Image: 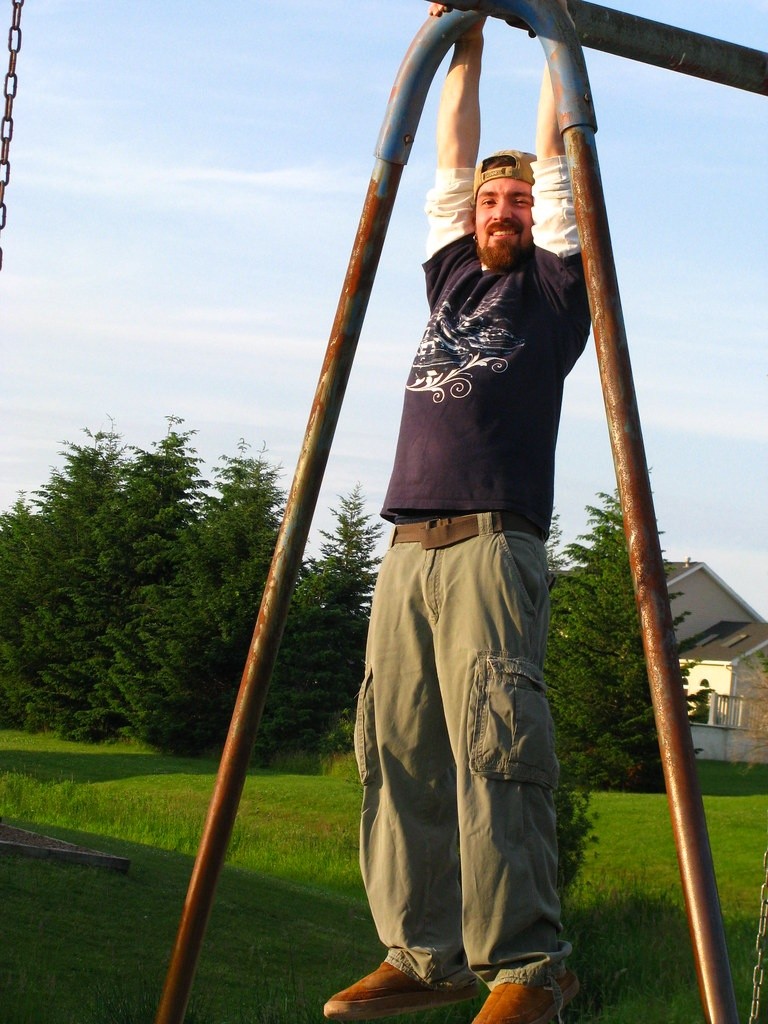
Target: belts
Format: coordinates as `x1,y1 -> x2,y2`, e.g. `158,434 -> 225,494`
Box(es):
392,516 -> 544,550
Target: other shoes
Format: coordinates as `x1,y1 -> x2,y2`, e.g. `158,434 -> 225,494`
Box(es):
471,967 -> 580,1024
324,963 -> 479,1020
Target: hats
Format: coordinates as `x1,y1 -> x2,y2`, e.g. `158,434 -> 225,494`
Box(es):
472,150 -> 537,204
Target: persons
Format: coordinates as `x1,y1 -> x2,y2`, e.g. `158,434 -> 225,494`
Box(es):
324,0 -> 591,1024
